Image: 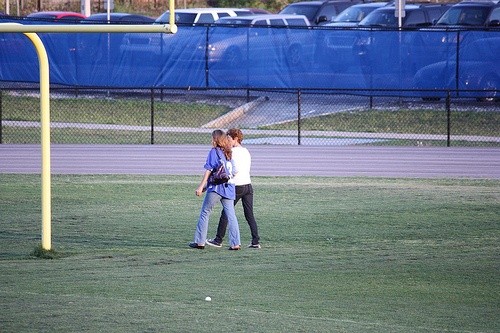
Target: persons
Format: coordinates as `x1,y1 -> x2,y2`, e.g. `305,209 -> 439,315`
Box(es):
188,130 -> 241,250
205,128 -> 261,248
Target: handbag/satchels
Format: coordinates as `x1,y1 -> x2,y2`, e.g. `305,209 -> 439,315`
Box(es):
209,148 -> 230,186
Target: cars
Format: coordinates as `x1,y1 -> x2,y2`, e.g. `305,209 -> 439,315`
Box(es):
120,8 -> 274,67
419,0 -> 500,102
80,13 -> 156,66
1,12 -> 86,64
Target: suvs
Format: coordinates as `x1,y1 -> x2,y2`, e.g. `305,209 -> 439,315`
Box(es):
171,14 -> 313,70
278,0 -> 453,72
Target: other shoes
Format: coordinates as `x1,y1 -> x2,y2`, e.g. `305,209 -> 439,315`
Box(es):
247,243 -> 260,249
206,239 -> 223,247
189,242 -> 204,249
228,247 -> 241,250
